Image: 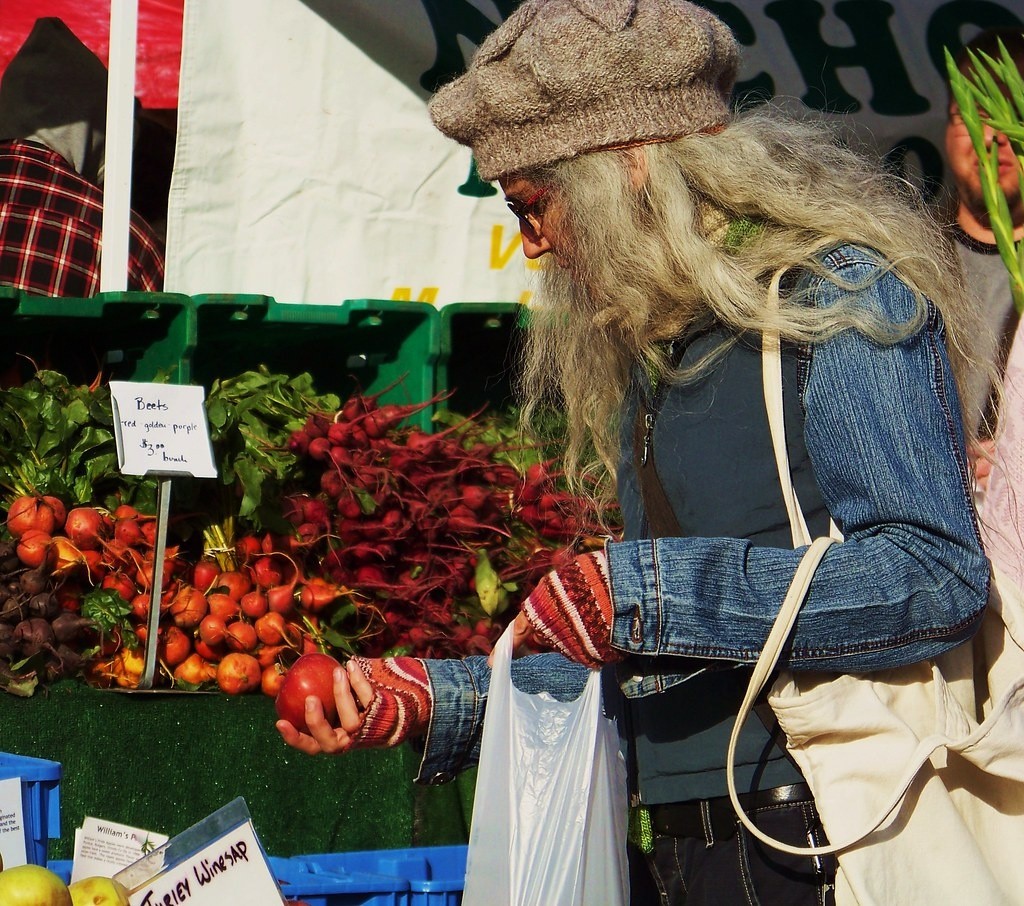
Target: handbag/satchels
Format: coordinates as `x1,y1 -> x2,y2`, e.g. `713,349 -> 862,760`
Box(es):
460,620 -> 631,906
767,609 -> 1024,906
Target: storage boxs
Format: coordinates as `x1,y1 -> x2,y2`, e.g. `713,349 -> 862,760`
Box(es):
0,751 -> 468,906
0,284 -> 575,442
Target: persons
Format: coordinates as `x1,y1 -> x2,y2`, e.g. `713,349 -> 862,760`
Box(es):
278,0 -> 993,905
925,0 -> 1024,519
0,17 -> 166,295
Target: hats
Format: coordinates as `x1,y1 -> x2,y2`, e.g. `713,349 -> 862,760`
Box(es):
428,0 -> 742,179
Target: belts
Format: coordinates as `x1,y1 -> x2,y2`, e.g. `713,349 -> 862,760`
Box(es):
650,782 -> 814,842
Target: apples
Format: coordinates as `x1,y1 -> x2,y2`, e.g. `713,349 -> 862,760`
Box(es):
275,653 -> 347,728
0,852 -> 128,906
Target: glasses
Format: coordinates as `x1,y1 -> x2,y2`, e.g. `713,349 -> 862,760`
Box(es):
507,181 -> 557,228
